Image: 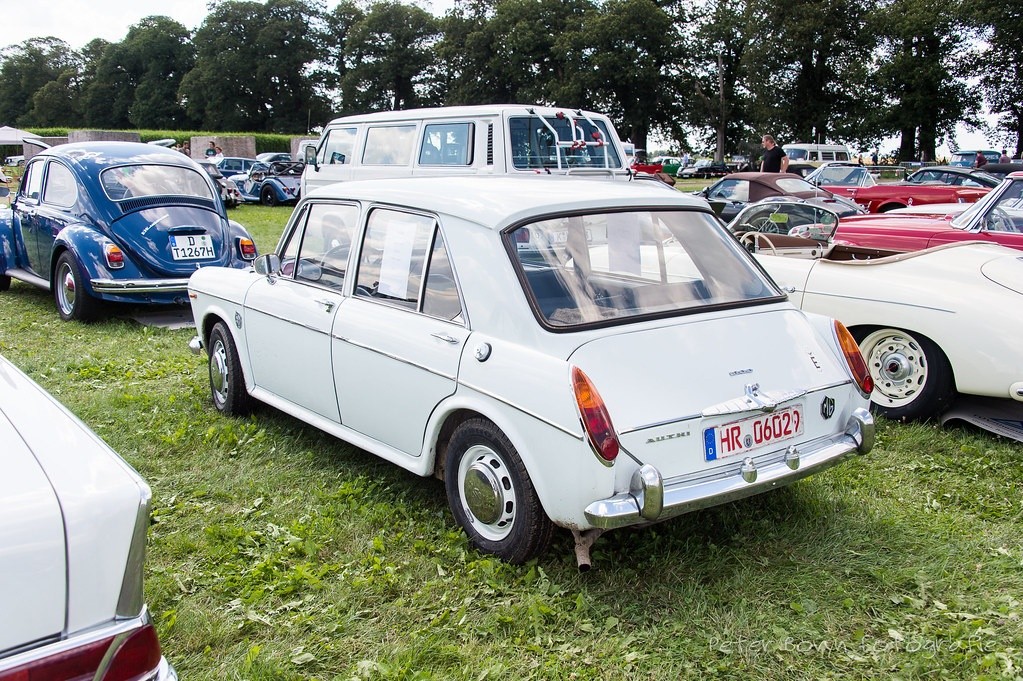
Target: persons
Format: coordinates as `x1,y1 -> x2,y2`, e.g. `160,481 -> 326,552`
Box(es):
681,150 -> 688,168
999,150 -> 1011,164
872,151 -> 881,179
974,149 -> 989,169
858,154 -> 865,168
759,135 -> 789,174
169,139 -> 226,160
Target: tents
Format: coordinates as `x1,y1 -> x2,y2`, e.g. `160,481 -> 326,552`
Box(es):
0,125 -> 44,167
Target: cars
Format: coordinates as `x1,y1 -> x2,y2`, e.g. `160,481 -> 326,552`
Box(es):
725,200 -> 1023,424
0,140 -> 261,321
620,140 -> 756,186
901,166 -> 1001,189
188,172 -> 875,560
803,160 -> 993,215
829,171 -> 1023,260
193,139 -> 321,209
685,172 -> 865,234
948,150 -> 1002,168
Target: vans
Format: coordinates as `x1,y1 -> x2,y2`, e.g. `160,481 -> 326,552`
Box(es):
300,105 -> 634,200
782,144 -> 850,169
